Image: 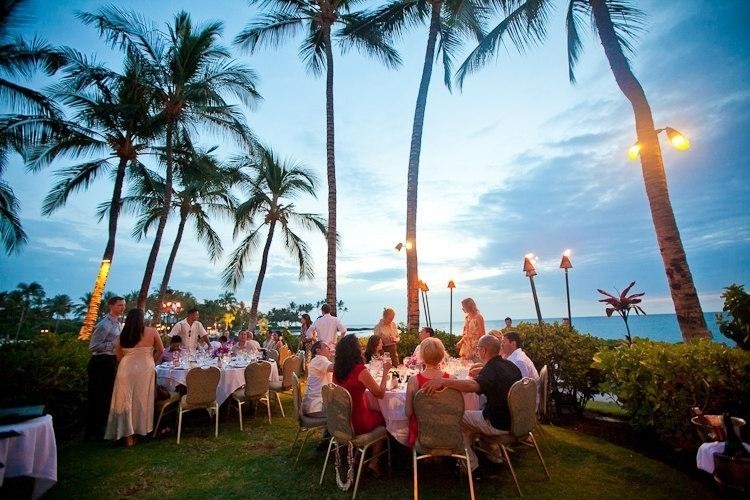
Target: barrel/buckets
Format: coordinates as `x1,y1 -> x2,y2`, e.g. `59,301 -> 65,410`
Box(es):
689,409 -> 746,444
4,404 -> 46,416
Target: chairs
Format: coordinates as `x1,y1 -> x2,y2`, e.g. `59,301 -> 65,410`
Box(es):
293,347 -> 549,500
154,348 -> 306,444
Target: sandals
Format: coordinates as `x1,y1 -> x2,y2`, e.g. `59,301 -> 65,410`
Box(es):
156,427 -> 171,436
124,435 -> 138,450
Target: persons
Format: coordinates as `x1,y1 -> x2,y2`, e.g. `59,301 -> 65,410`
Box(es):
87,296 -> 126,440
562,318 -> 569,327
103,308 -> 164,449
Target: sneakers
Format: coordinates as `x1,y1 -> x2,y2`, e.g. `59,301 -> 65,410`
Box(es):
473,466 -> 498,480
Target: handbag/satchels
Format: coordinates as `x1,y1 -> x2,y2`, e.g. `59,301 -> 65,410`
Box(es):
157,384 -> 171,402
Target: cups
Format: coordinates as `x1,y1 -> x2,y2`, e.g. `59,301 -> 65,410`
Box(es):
384,352 -> 390,366
157,347 -> 269,369
366,357 -> 474,388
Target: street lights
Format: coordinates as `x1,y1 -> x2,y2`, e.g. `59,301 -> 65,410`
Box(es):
519,248 -> 577,329
625,123 -> 692,159
393,241 -> 456,344
161,300 -> 183,328
